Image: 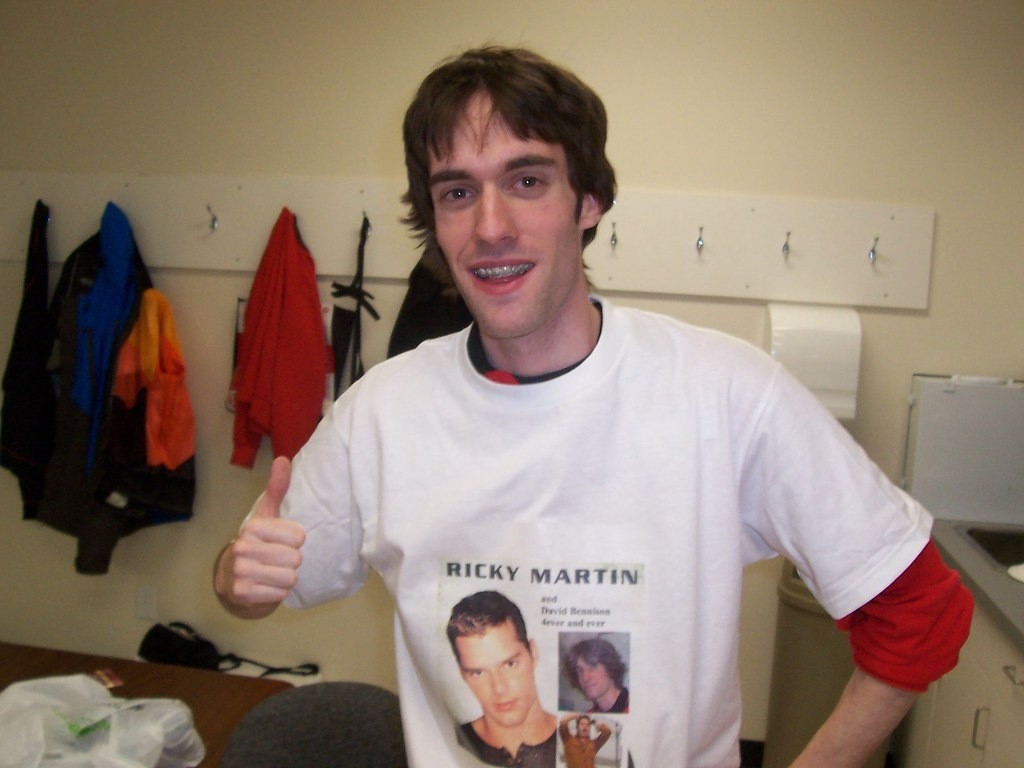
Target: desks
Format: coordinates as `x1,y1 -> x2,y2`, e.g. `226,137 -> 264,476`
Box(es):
1,643 -> 296,768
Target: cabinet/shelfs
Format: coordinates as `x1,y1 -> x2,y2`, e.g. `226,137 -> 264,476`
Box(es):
892,596 -> 1024,768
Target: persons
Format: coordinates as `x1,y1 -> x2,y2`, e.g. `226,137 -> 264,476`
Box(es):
209,43 -> 977,767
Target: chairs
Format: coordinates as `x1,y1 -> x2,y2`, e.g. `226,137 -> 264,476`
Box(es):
217,681 -> 409,768
